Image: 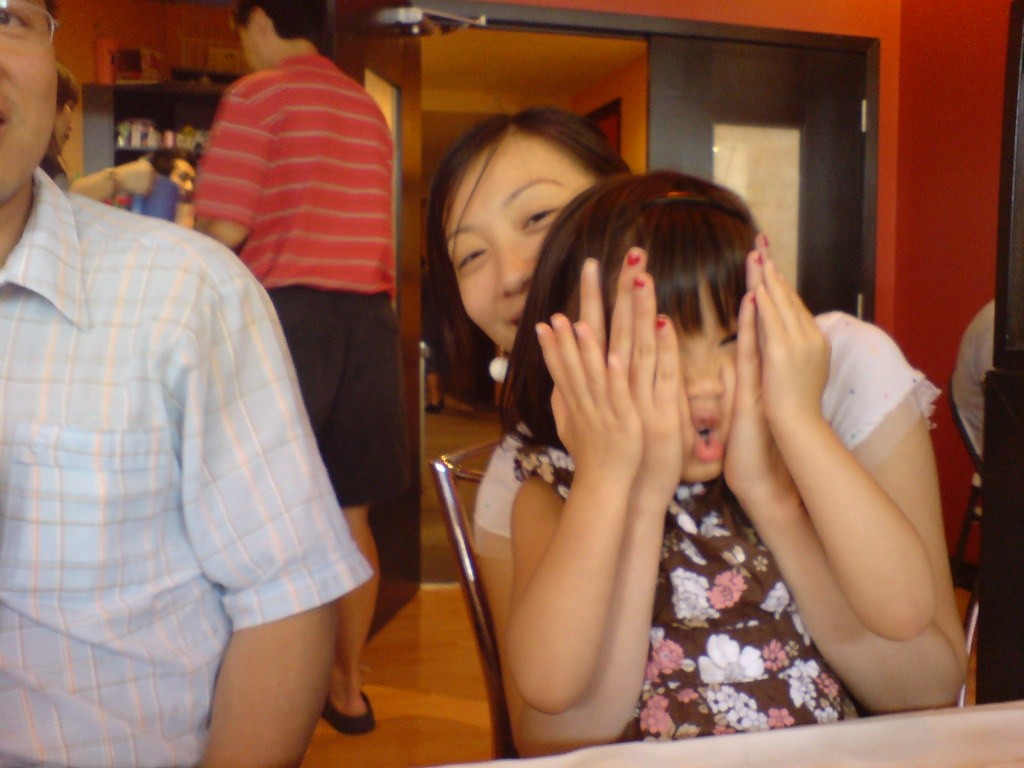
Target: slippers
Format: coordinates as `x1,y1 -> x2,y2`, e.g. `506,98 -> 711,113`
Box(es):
323,691 -> 376,733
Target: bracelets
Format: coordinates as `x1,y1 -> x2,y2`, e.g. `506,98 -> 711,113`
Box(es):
103,167 -> 119,198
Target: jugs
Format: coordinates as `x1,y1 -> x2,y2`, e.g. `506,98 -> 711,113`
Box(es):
131,175 -> 179,221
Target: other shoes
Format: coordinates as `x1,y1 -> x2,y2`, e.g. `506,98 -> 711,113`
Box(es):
425,403 -> 441,412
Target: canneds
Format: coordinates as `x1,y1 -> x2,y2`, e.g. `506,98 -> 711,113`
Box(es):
163,128 -> 177,148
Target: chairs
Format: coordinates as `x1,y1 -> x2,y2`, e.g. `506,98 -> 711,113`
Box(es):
430,374 -> 984,760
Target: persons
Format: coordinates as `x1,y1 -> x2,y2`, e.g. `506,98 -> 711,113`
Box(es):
420,105 -> 969,759
0,1 -> 376,768
39,59 -> 157,203
193,0 -> 408,736
154,146 -> 197,204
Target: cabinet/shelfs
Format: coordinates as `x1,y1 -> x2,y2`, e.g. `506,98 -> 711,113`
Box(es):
80,82 -> 229,228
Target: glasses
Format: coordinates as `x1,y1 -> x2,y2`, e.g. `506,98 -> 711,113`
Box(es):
0,1 -> 56,45
